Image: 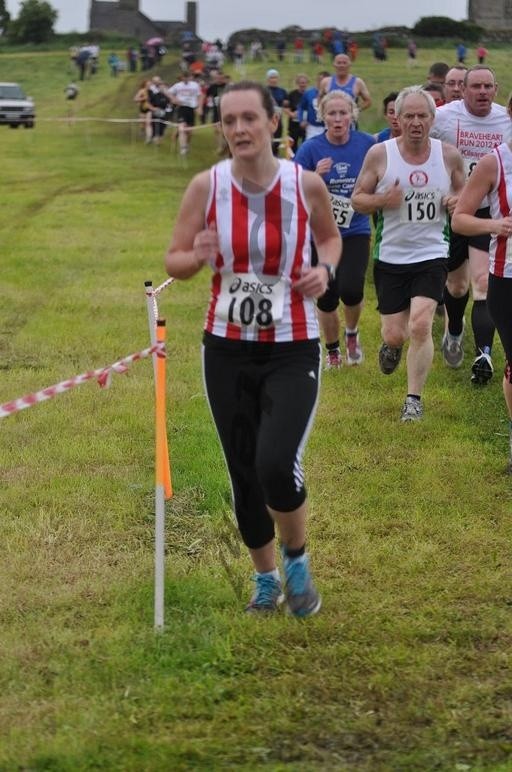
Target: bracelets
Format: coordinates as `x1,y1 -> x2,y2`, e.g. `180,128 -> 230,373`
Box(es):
317,262 -> 336,282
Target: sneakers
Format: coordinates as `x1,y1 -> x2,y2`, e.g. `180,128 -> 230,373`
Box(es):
138,133 -> 195,159
325,341 -> 343,372
398,395 -> 424,427
282,542 -> 323,620
343,328 -> 363,367
470,353 -> 495,386
378,341 -> 402,375
442,316 -> 466,369
242,565 -> 285,617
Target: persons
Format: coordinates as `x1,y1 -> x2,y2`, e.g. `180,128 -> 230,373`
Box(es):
422,62 -> 512,387
261,70 -> 287,156
63,78 -> 81,102
296,71 -> 332,140
292,90 -> 379,377
371,90 -> 403,143
287,73 -> 309,155
132,65 -> 236,158
181,34 -> 419,73
476,43 -> 488,64
453,39 -> 468,65
449,91 -> 512,417
68,37 -> 166,78
349,86 -> 466,426
163,80 -> 343,626
318,53 -> 372,131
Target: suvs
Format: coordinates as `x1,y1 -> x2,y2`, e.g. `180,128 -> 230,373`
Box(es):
0,81 -> 36,130
